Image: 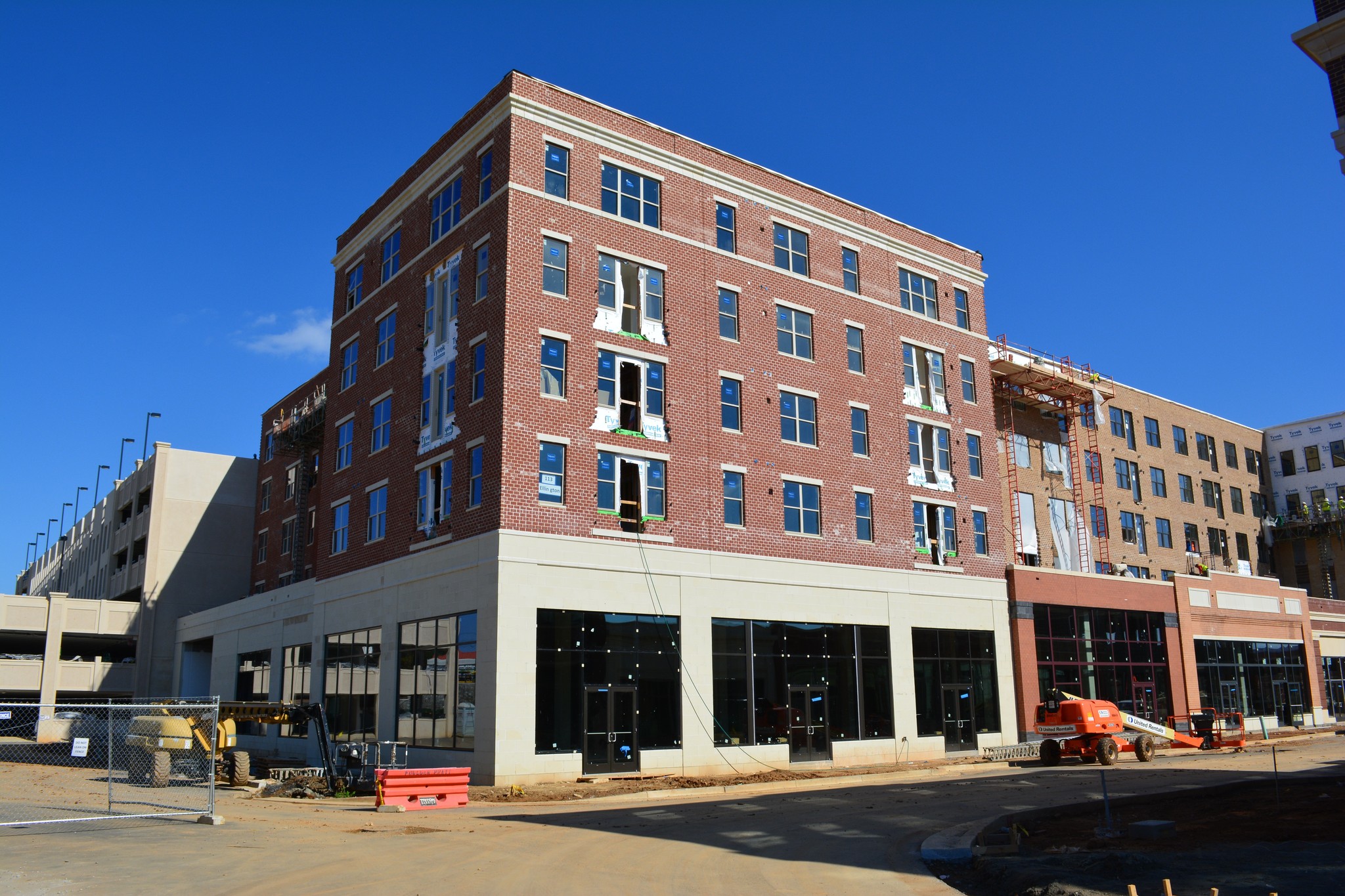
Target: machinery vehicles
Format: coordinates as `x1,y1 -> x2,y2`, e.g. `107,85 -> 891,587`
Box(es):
1033,688 -> 1246,767
124,699 -> 408,793
